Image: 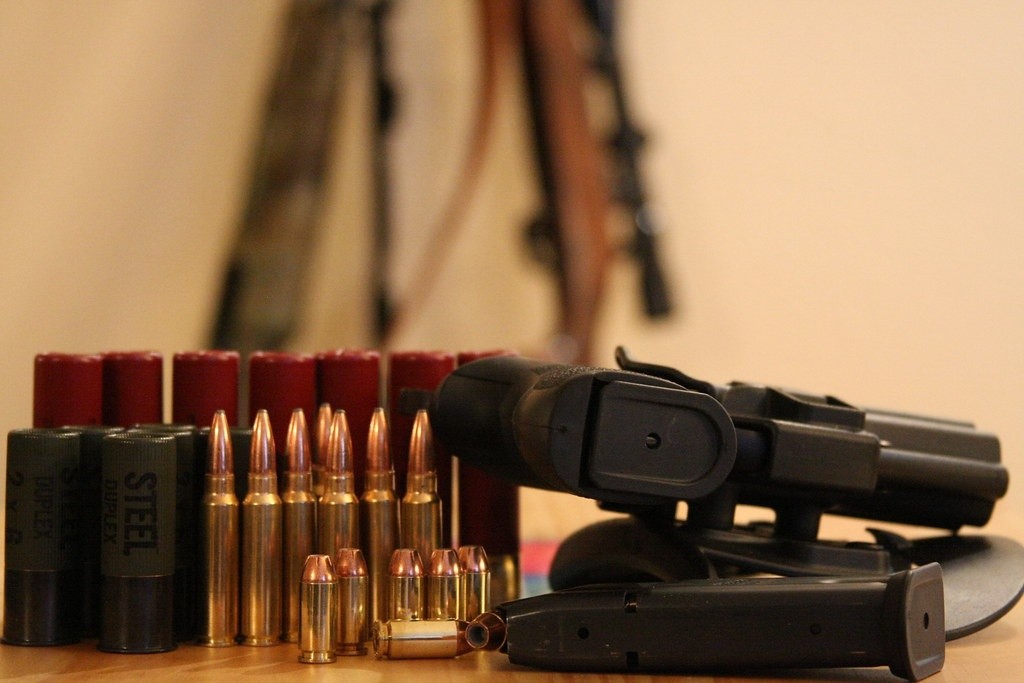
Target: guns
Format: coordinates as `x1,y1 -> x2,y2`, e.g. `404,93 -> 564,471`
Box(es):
200,0 -> 679,364
398,346 -> 1008,544
